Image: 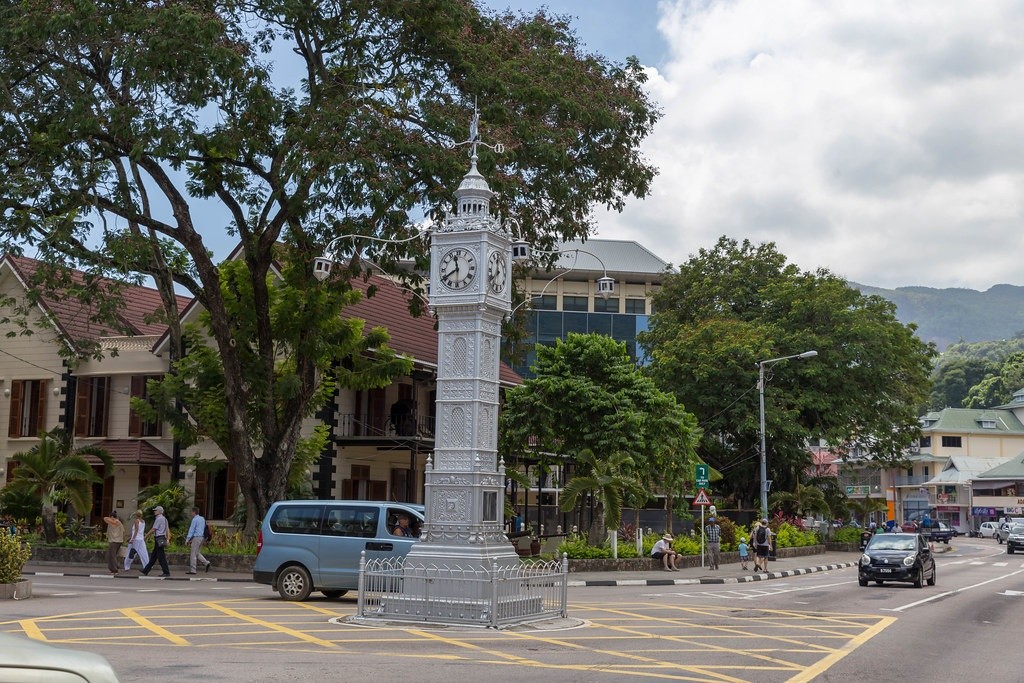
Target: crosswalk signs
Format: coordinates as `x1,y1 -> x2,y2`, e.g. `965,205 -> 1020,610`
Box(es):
692,489 -> 712,505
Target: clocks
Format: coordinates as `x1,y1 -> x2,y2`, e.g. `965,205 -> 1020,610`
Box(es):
487,250 -> 508,294
438,248 -> 477,289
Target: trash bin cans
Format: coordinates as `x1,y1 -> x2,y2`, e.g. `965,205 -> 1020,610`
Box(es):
860,532 -> 872,552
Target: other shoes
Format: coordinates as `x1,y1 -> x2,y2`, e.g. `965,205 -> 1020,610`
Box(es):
715,563 -> 718,569
671,567 -> 680,571
754,564 -> 769,573
158,573 -> 170,577
109,569 -> 119,574
139,569 -> 147,576
664,566 -> 672,572
205,564 -> 210,573
742,566 -> 748,570
709,566 -> 714,570
185,571 -> 196,574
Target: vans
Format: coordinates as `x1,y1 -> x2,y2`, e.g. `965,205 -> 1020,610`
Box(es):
252,500 -> 427,602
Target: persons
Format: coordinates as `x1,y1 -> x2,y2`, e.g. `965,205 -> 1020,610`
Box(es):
932,519 -> 939,528
185,507 -> 210,574
738,537 -> 750,570
753,519 -> 773,573
668,533 -> 682,569
123,510 -> 150,571
137,506 -> 171,577
881,522 -> 888,532
517,513 -> 522,532
104,512 -> 123,575
392,514 -> 415,538
749,522 -> 762,571
705,518 -> 721,570
891,522 -> 903,532
651,534 -> 680,571
922,513 -> 932,527
875,525 -> 884,534
912,518 -> 921,533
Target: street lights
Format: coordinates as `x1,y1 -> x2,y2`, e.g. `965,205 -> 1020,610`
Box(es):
760,350 -> 817,524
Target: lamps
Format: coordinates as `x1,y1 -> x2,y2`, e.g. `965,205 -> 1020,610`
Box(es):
119,468 -> 125,477
305,470 -> 311,477
0,468 -> 4,475
4,389 -> 10,396
123,386 -> 128,394
52,388 -> 59,396
187,469 -> 193,477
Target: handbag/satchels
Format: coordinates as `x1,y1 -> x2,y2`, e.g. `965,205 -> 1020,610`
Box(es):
129,548 -> 137,559
155,536 -> 167,547
117,547 -> 126,557
750,531 -> 754,547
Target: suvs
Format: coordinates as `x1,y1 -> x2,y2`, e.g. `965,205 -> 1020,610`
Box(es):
1006,524 -> 1024,554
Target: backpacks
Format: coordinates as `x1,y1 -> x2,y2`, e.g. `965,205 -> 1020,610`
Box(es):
202,516 -> 212,541
756,526 -> 767,544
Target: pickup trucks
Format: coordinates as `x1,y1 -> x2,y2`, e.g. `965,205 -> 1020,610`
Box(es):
995,522 -> 1021,544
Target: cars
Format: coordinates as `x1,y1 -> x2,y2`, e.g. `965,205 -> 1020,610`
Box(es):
814,519 -> 842,527
979,522 -> 999,539
858,532 -> 936,588
901,520 -> 954,544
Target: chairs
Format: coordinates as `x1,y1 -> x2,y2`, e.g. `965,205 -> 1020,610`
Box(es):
388,515 -> 398,532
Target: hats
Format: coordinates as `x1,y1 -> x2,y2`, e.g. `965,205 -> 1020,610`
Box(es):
762,519 -> 769,523
135,510 -> 143,517
709,517 -> 715,523
152,505 -> 164,513
398,513 -> 409,521
662,534 -> 673,541
740,537 -> 746,542
755,522 -> 762,527
